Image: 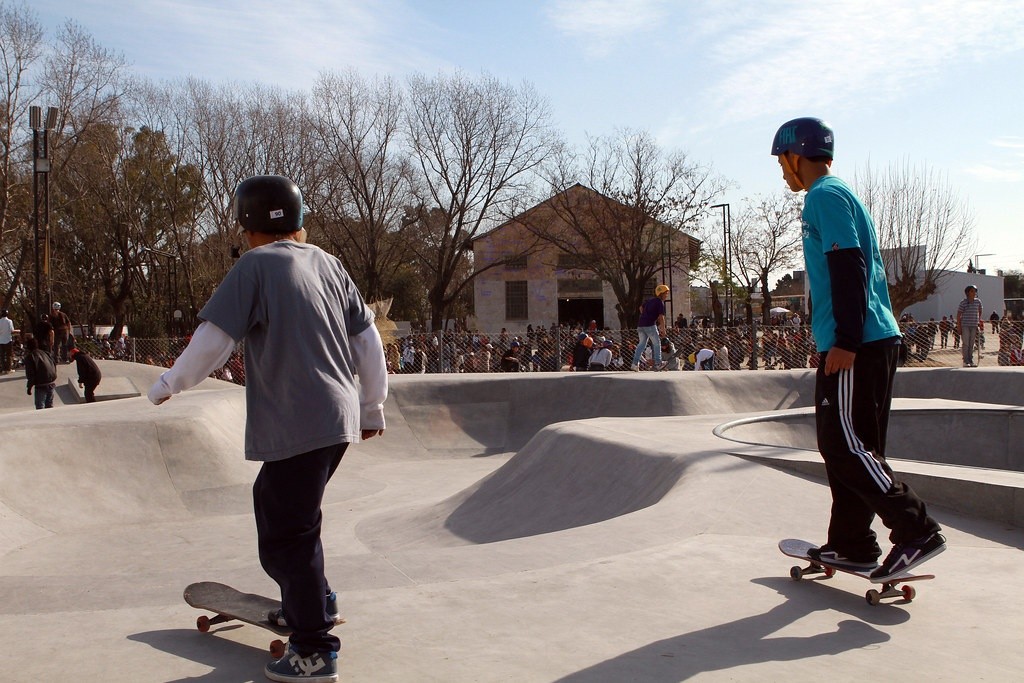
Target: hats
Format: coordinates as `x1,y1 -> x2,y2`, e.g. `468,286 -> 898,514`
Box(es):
592,320 -> 596,323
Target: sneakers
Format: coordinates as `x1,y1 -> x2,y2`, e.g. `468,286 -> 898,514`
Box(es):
264,650 -> 340,683
267,590 -> 339,625
806,540 -> 878,569
868,531 -> 947,584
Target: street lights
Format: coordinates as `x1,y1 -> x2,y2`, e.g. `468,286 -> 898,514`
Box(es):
29,106 -> 58,320
710,203 -> 735,328
975,254 -> 996,272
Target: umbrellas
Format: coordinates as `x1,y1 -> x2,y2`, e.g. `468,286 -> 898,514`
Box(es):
770,306 -> 790,313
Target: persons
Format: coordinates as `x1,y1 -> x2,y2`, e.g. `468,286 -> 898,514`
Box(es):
71,348 -> 102,403
898,312 -> 928,360
0,309 -> 21,374
147,176 -> 388,682
33,314 -> 54,353
11,334 -> 245,386
48,302 -> 71,364
771,117 -> 948,586
381,313 -> 820,373
990,310 -> 999,333
25,338 -> 57,410
631,285 -> 670,371
998,314 -> 1024,366
928,314 -> 985,350
957,285 -> 983,367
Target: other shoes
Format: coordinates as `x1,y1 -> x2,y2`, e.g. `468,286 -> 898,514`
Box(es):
1,369 -> 15,375
65,359 -> 70,363
963,362 -> 978,367
656,360 -> 669,370
631,364 -> 639,372
55,359 -> 60,363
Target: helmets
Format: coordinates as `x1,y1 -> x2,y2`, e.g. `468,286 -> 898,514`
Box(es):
583,336 -> 593,347
52,301 -> 63,311
233,175 -> 303,235
1,309 -> 9,316
511,341 -> 520,347
771,117 -> 834,163
485,344 -> 493,349
603,340 -> 613,348
70,348 -> 79,358
655,284 -> 670,297
660,337 -> 669,347
579,332 -> 589,340
688,352 -> 696,364
41,313 -> 48,320
965,285 -> 978,295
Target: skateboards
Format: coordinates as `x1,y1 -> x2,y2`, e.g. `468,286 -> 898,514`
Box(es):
654,345 -> 684,372
977,326 -> 984,366
778,538 -> 936,606
183,580 -> 348,657
